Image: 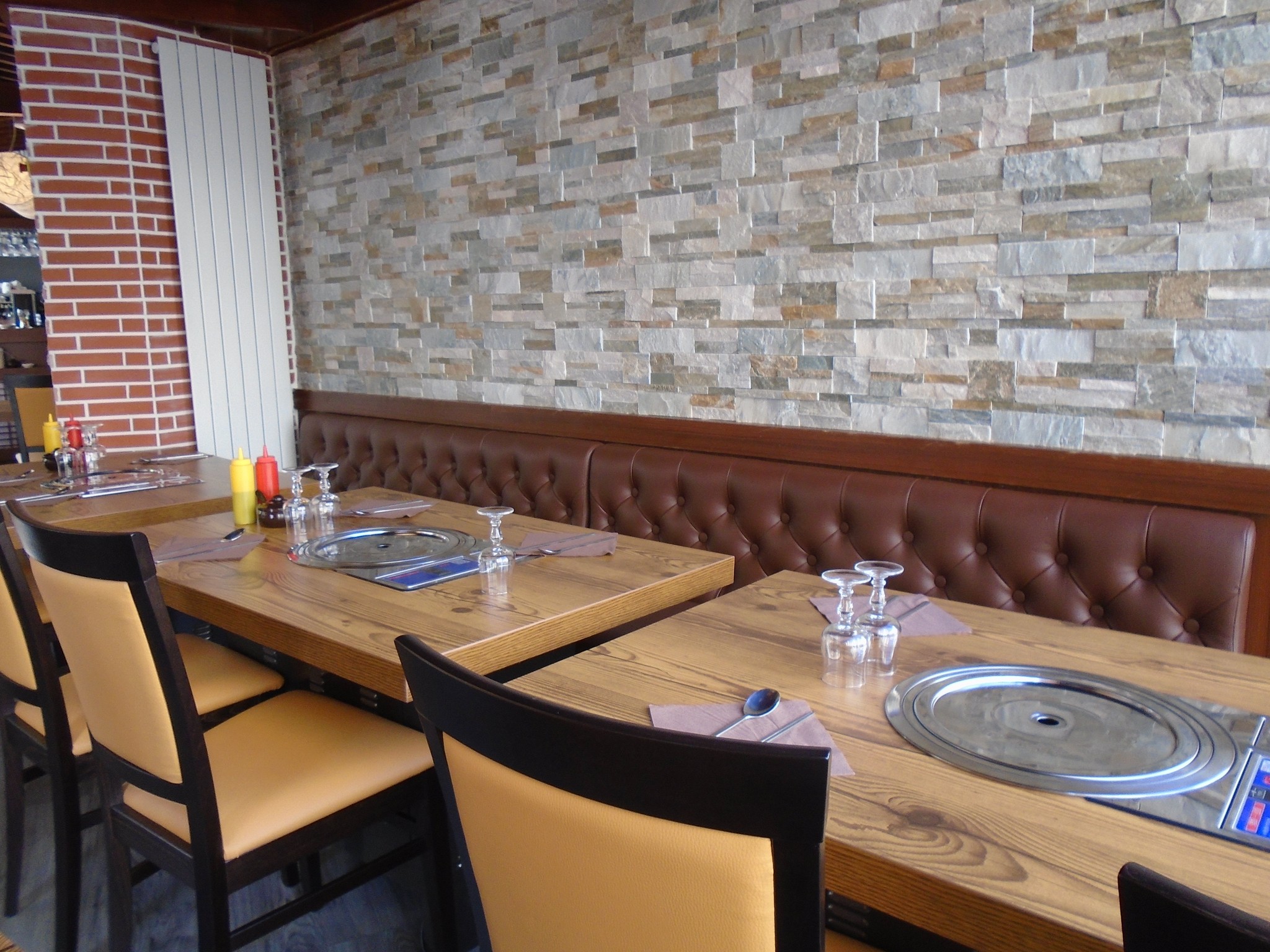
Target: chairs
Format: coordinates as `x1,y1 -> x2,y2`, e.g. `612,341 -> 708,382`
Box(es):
7,499 -> 462,952
2,374 -> 57,462
0,511 -> 284,951
396,634 -> 831,951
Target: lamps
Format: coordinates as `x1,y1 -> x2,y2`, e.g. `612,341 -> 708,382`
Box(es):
0,115 -> 33,205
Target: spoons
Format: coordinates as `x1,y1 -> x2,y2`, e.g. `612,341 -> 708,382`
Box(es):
14,469 -> 35,479
153,528 -> 246,556
711,688 -> 780,737
350,504 -> 432,516
538,537 -> 614,555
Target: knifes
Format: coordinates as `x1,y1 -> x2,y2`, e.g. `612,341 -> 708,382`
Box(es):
154,540 -> 260,563
515,532 -> 599,552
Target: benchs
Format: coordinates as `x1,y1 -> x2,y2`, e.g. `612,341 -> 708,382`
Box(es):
289,389 -> 1270,657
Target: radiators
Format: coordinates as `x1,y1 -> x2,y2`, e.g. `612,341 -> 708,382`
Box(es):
154,37 -> 298,472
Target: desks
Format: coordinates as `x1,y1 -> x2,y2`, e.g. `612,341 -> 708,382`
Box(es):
0,452 -> 322,550
129,485 -> 734,702
500,569 -> 1270,951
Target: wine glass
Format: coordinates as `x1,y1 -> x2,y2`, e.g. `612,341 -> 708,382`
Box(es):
477,506 -> 514,595
820,569 -> 871,688
309,463 -> 341,531
53,424 -> 105,478
852,560 -> 903,678
281,466 -> 312,536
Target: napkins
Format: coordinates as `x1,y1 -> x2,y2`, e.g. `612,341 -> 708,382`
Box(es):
647,698 -> 855,778
131,453 -> 214,465
3,490 -> 81,506
515,532 -> 619,556
341,500 -> 438,519
809,593 -> 972,636
0,474 -> 54,486
152,533 -> 267,561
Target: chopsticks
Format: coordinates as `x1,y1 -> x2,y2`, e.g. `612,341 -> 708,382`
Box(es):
760,711 -> 815,743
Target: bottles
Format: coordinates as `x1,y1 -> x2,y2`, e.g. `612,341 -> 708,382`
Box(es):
229,447 -> 257,525
42,413 -> 61,454
64,413 -> 82,450
255,445 -> 280,504
